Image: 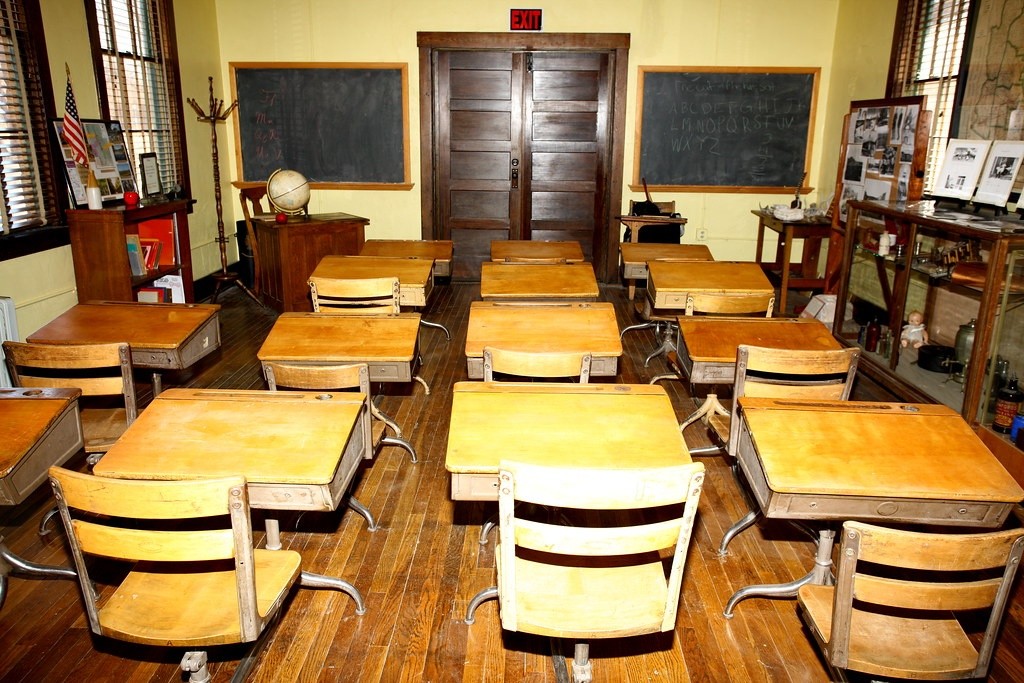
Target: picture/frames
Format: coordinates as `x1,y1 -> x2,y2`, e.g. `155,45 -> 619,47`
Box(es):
933,139 -> 992,200
973,139 -> 1024,207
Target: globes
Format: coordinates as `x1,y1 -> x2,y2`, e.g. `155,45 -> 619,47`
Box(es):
266,167 -> 310,223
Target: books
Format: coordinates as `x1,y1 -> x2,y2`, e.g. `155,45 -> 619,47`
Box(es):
125,234 -> 164,276
137,287 -> 172,303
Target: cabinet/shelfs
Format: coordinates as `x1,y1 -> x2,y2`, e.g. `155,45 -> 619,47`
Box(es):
65,198 -> 194,304
832,198 -> 1024,518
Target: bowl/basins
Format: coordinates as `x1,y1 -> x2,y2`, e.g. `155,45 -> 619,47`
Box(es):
918,344 -> 961,372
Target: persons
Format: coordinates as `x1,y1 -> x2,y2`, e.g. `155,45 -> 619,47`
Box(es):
900,311 -> 929,349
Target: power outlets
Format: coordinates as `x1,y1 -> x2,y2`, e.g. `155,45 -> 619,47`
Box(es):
697,229 -> 708,241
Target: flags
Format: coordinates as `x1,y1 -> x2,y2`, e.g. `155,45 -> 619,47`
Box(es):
62,76 -> 90,170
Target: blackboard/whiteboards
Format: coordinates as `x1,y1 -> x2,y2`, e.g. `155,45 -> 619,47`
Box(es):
229,61 -> 415,191
628,65 -> 822,195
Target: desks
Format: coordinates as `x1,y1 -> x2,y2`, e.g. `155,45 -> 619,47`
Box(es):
618,242 -> 714,338
722,396 -> 1024,620
492,239 -> 585,263
26,301 -> 222,398
645,261 -> 774,367
93,388 -> 367,615
480,261 -> 600,302
257,312 -> 422,439
306,255 -> 436,365
465,302 -> 622,379
359,239 -> 454,341
751,211 -> 830,314
671,315 -> 845,433
0,387 -> 102,610
258,210 -> 369,314
444,381 -> 695,683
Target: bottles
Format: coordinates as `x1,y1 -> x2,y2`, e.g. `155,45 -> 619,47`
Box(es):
878,230 -> 890,256
976,354 -> 1024,452
905,171 -> 924,210
896,173 -> 908,210
954,324 -> 975,383
857,314 -> 894,363
86,170 -> 98,187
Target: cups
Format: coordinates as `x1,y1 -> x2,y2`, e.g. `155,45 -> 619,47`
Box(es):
86,188 -> 102,209
889,234 -> 897,246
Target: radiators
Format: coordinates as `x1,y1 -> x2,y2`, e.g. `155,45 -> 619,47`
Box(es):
0,296 -> 18,388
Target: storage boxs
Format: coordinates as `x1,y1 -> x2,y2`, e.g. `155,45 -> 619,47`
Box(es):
815,295 -> 853,324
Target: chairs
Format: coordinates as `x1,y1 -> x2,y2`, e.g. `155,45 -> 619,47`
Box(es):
0,185 -> 1024,683
